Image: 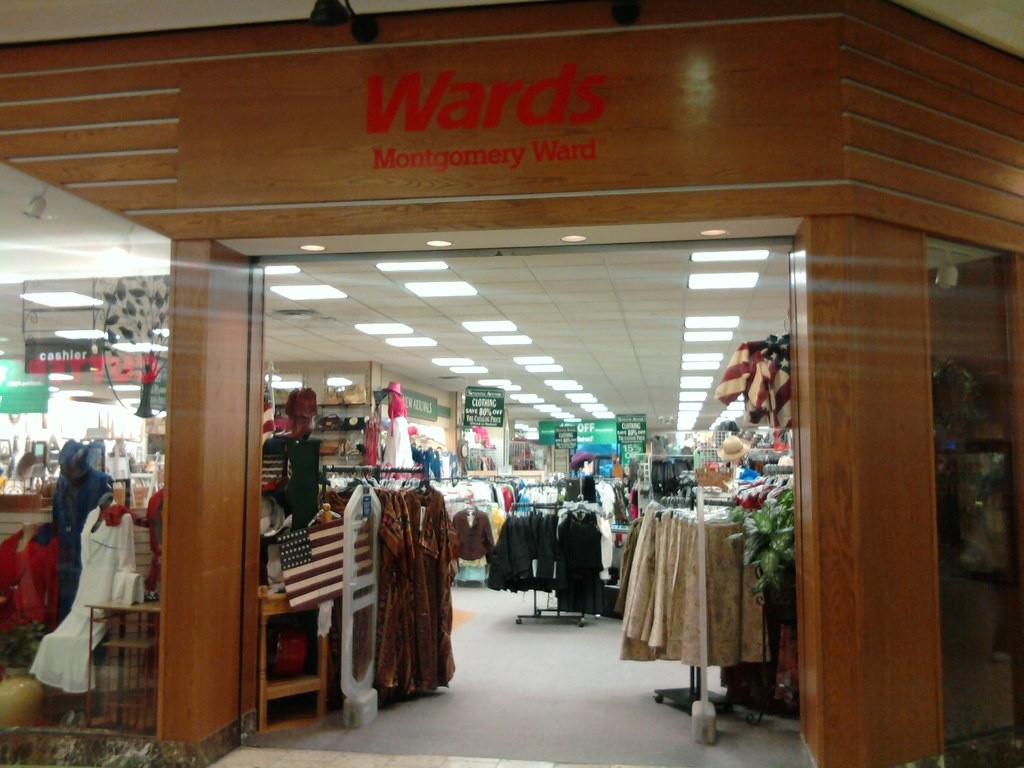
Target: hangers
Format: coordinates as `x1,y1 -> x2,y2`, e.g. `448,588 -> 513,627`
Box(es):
734,464 -> 796,501
635,497 -> 735,528
510,494 -> 609,521
329,462 -> 438,494
433,477 -> 520,513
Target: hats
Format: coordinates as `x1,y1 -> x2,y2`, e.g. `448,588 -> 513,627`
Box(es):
384,380 -> 403,396
569,451 -> 595,470
58,439 -> 104,478
717,437 -> 749,460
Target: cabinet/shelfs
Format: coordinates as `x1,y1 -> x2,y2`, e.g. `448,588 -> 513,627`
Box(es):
315,402 -> 368,456
86,600 -> 159,728
258,585 -> 329,731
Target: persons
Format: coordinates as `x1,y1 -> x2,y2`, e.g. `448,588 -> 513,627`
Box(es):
102,487 -> 163,598
31,440 -> 115,666
1,452 -> 36,497
0,513 -> 60,646
29,490 -> 136,726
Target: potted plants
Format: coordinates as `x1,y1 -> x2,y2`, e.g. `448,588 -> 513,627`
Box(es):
730,489 -> 797,614
0,621 -> 52,727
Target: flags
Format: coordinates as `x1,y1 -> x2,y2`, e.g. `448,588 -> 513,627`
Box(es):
262,368 -> 276,492
278,518 -> 374,608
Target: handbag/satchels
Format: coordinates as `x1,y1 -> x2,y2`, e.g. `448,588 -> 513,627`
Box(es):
343,382 -> 365,404
272,408 -> 289,431
319,438 -> 346,456
342,417 -> 365,431
317,414 -> 340,430
321,384 -> 342,406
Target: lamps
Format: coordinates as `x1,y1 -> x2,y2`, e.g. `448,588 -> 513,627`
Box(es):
307,1 -> 379,42
934,241 -> 959,289
21,183 -> 54,219
